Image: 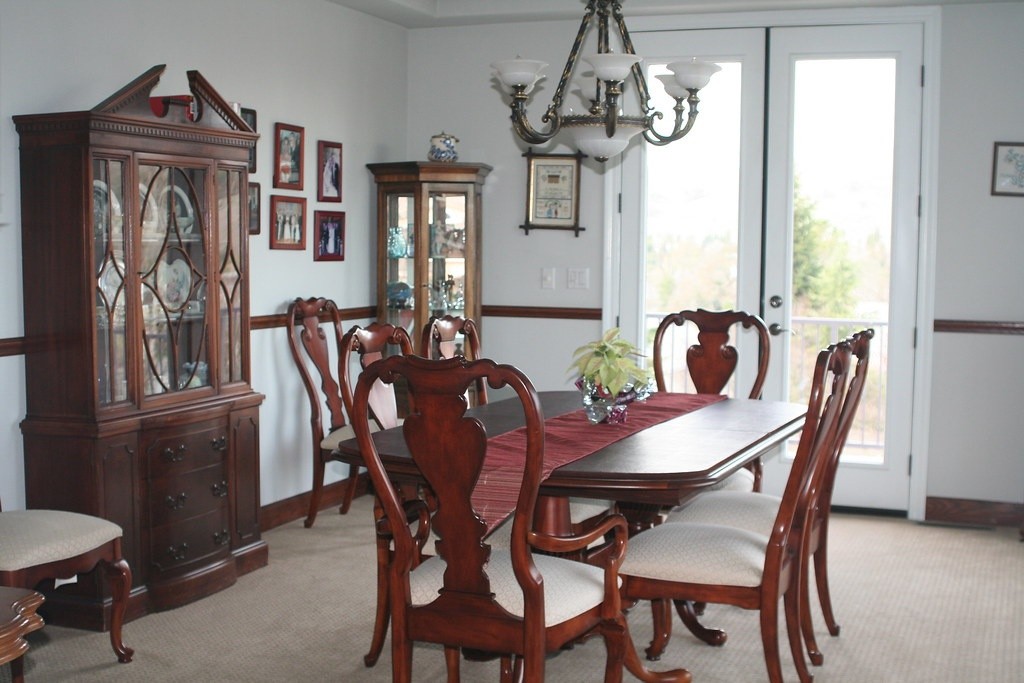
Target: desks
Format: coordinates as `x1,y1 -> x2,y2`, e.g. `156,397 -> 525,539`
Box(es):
338,390 -> 809,683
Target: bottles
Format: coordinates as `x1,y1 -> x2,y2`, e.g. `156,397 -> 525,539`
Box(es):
386,227 -> 406,257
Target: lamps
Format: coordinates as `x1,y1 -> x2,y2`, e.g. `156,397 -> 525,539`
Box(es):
490,0 -> 722,163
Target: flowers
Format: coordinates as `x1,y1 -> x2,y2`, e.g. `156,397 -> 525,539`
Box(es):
571,327 -> 654,400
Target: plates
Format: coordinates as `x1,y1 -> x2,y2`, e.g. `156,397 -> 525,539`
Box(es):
91,179 -> 193,311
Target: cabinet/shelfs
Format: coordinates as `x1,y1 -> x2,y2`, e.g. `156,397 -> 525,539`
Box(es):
13,64 -> 267,635
366,160 -> 493,410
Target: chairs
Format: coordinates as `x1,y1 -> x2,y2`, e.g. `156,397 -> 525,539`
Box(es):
617,335 -> 853,683
340,322 -> 515,668
650,327 -> 875,664
653,309 -> 772,492
423,315 -> 489,405
287,296 -> 406,528
353,356 -> 629,683
0,500 -> 134,663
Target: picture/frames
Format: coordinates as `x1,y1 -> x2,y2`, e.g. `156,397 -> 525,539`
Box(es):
240,108 -> 345,261
991,141 -> 1024,197
518,146 -> 588,237
